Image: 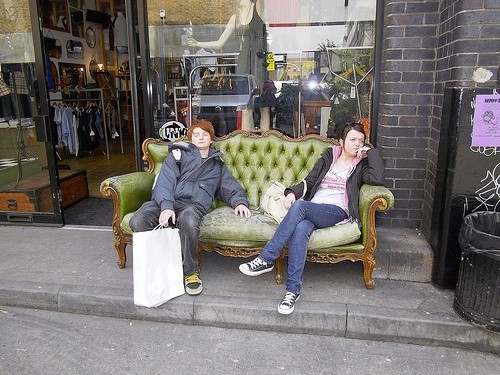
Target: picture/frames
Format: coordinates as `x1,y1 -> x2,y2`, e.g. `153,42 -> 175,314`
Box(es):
68,0 -> 84,10
38,0 -> 71,33
70,7 -> 85,39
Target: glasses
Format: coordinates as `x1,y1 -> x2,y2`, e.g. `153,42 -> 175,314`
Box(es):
341,122 -> 364,134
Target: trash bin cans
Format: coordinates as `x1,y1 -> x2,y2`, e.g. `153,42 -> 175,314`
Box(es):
452,210 -> 500,334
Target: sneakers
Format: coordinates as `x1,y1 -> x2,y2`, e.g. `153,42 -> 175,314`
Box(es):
278,290 -> 301,315
186,270 -> 202,295
238,257 -> 274,276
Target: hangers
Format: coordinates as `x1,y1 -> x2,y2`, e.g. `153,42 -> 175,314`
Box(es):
51,99 -> 112,113
198,66 -> 233,90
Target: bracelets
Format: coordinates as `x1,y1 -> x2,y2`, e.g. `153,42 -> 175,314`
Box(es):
318,90 -> 322,94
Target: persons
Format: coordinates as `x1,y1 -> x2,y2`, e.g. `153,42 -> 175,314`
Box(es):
187,0 -> 277,130
259,80 -> 278,127
129,119 -> 252,295
239,123 -> 385,314
303,73 -> 329,101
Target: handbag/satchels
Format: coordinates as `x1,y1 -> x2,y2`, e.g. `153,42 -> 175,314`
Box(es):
132,222 -> 186,308
262,180 -> 304,222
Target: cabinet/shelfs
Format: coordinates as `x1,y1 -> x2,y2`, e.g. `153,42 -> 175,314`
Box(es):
96,0 -> 126,76
115,75 -> 134,137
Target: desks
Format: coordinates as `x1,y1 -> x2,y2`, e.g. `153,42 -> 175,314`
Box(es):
293,100 -> 332,139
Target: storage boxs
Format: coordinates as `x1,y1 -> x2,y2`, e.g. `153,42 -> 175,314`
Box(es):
0,168 -> 91,218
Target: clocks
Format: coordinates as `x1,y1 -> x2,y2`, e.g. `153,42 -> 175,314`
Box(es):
85,26 -> 97,49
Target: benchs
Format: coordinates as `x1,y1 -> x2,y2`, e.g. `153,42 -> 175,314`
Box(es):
99,128 -> 395,290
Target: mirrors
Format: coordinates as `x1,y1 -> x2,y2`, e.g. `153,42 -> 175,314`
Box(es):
66,40 -> 85,59
58,61 -> 87,107
